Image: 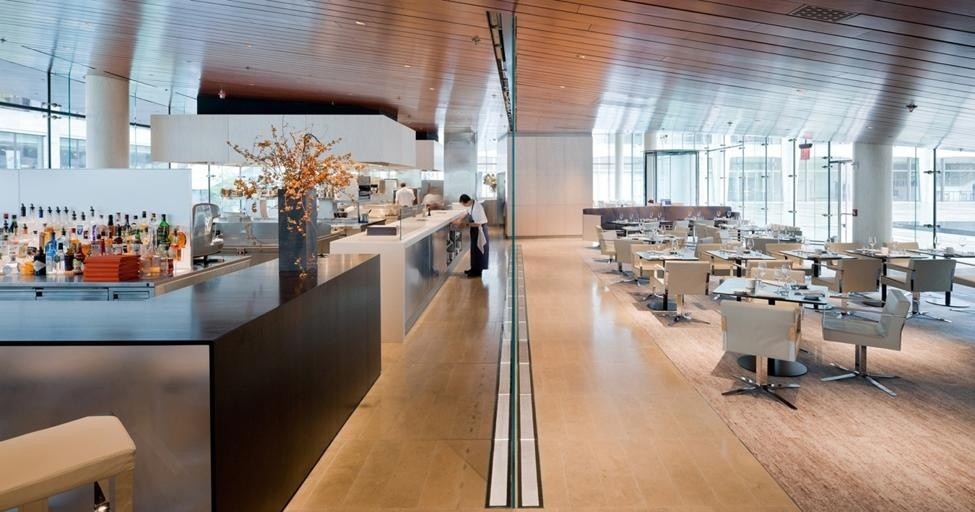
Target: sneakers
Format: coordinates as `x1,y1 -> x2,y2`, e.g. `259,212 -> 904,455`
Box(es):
465,270 -> 481,277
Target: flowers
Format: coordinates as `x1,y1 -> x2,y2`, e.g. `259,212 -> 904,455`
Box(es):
214,111 -> 386,279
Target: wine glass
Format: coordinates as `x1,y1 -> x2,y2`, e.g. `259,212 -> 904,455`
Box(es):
774,266 -> 783,287
618,212 -> 634,222
716,210 -> 742,221
796,235 -> 831,252
721,237 -> 755,254
762,224 -> 791,239
648,237 -> 678,254
934,236 -> 942,249
782,263 -> 792,286
638,222 -> 660,236
688,211 -> 702,219
959,237 -> 967,252
869,235 -> 876,253
758,261 -> 768,287
649,211 -> 663,218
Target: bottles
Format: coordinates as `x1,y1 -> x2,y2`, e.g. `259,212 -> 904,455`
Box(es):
0,203 -> 179,276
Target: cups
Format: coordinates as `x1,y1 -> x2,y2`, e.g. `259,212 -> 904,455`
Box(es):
745,278 -> 755,288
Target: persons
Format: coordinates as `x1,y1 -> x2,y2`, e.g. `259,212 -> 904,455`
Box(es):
395,182 -> 416,208
454,193 -> 489,278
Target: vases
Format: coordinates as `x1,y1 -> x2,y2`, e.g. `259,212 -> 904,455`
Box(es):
274,188 -> 319,278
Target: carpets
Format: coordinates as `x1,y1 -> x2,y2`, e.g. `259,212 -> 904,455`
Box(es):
584,256 -> 973,512
